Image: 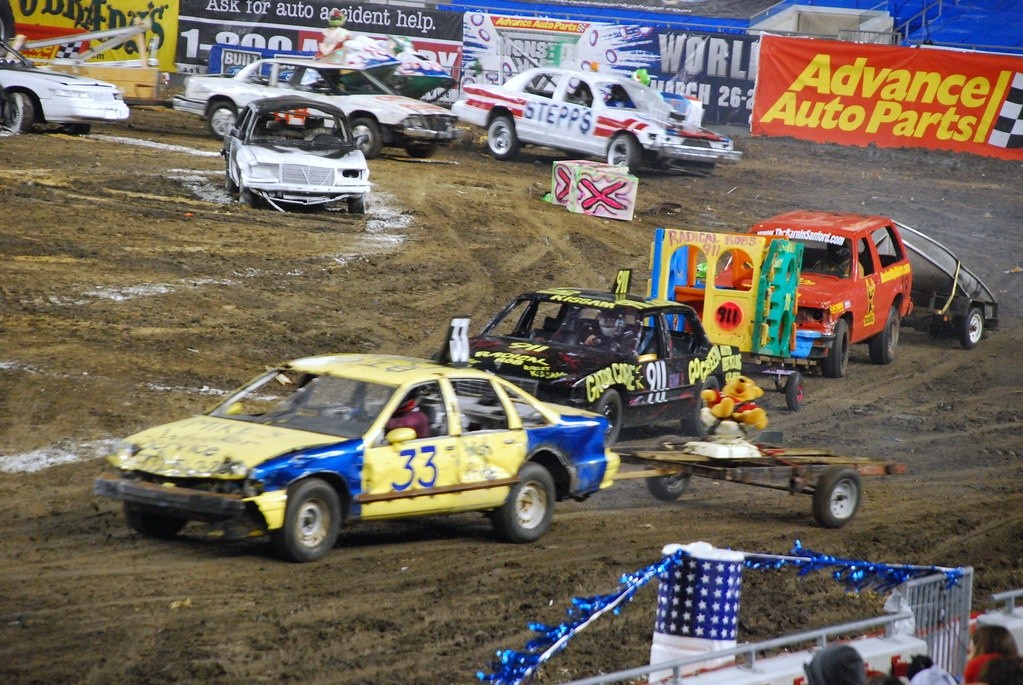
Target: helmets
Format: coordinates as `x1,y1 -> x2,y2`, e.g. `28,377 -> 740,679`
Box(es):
827,240 -> 850,263
612,85 -> 626,100
395,388 -> 420,413
598,310 -> 626,336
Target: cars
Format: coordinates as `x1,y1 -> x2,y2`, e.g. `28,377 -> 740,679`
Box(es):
447,268 -> 744,443
450,64 -> 743,173
91,314 -> 622,564
173,54 -> 460,159
215,93 -> 372,215
0,37 -> 132,135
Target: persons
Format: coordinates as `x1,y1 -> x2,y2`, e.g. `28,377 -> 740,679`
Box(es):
796,624 -> 1023,684
385,388 -> 430,437
605,85 -> 636,109
568,81 -> 593,108
584,310 -> 637,358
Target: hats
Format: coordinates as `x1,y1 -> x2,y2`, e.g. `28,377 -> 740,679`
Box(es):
804,646 -> 865,685
964,653 -> 1003,685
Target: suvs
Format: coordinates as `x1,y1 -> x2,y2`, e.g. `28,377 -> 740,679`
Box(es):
732,205 -> 915,378
